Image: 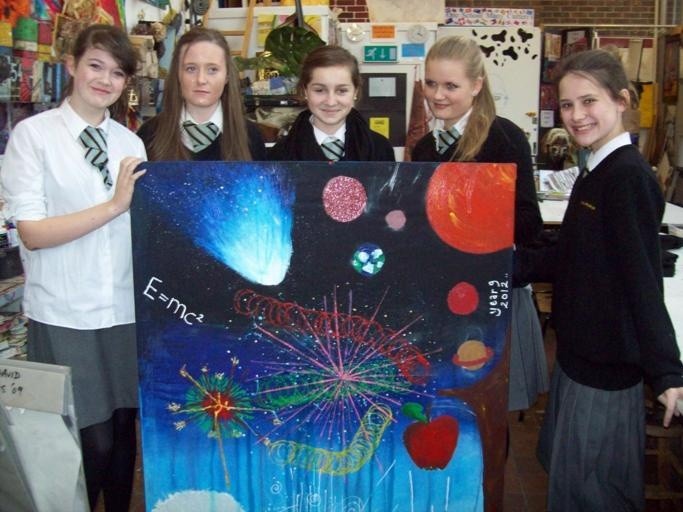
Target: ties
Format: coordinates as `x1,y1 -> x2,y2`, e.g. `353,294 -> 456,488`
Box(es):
320,139 -> 344,162
78,125 -> 113,192
183,121 -> 219,152
437,127 -> 460,155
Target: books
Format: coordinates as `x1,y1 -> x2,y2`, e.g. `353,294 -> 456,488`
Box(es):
1,277 -> 27,360
539,168 -> 579,197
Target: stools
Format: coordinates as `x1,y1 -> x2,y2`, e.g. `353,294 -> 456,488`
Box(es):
645,405 -> 680,509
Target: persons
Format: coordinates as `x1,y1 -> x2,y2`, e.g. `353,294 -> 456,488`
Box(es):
137,27 -> 269,160
536,48 -> 683,512
270,47 -> 395,161
412,36 -> 550,413
1,25 -> 148,512
